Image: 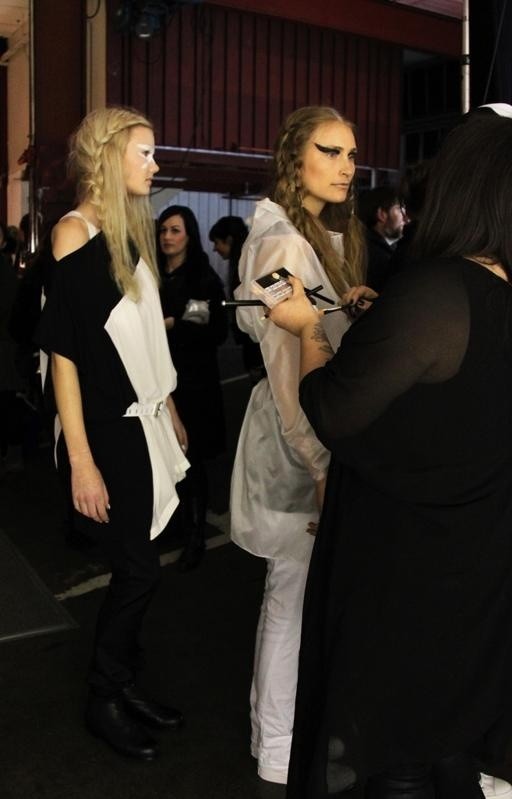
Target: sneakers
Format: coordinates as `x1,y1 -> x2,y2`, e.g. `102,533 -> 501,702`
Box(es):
251,735 -> 357,798
479,772 -> 512,799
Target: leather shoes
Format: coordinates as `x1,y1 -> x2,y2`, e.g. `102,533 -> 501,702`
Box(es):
84,704 -> 154,761
123,681 -> 176,724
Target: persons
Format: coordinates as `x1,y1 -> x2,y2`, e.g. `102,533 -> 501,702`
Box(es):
208,213 -> 267,401
228,104 -> 361,799
155,204 -> 230,578
268,100 -> 510,799
43,103 -> 192,761
357,185 -> 411,305
0,211 -> 44,480
